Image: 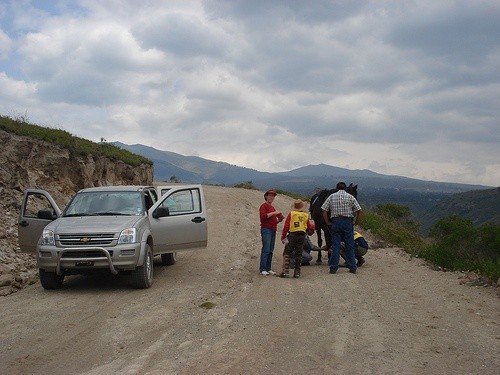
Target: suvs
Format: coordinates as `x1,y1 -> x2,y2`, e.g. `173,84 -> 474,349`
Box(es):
17,185 -> 209,290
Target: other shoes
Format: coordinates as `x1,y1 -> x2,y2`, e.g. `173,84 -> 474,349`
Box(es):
358,258 -> 365,266
262,271 -> 269,275
268,270 -> 276,275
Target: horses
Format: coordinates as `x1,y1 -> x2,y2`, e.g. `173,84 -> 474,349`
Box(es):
308,182 -> 359,266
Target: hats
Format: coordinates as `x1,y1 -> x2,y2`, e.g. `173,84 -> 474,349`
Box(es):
292,198 -> 307,211
265,190 -> 277,196
309,219 -> 317,230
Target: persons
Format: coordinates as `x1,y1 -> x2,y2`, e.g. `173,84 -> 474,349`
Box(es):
276,199 -> 311,277
321,181 -> 362,274
259,191 -> 285,276
330,229 -> 368,268
282,223 -> 326,269
